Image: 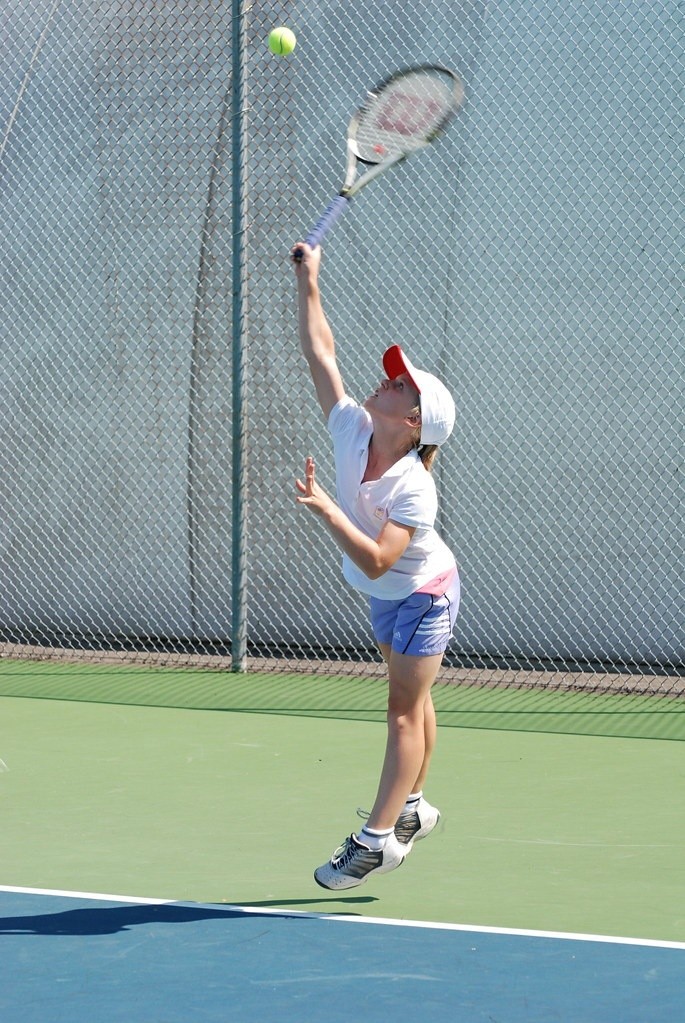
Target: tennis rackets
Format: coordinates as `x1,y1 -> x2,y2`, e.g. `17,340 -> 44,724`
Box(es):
290,59 -> 467,265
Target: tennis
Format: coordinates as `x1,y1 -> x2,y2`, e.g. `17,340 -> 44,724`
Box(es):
268,26 -> 299,56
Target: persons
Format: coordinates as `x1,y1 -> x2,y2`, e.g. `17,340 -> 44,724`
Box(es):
289,243 -> 462,890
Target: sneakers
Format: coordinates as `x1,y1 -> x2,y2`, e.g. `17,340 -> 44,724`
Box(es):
314,830 -> 405,890
357,796 -> 441,857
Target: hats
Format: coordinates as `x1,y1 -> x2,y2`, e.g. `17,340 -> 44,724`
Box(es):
383,345 -> 456,448
